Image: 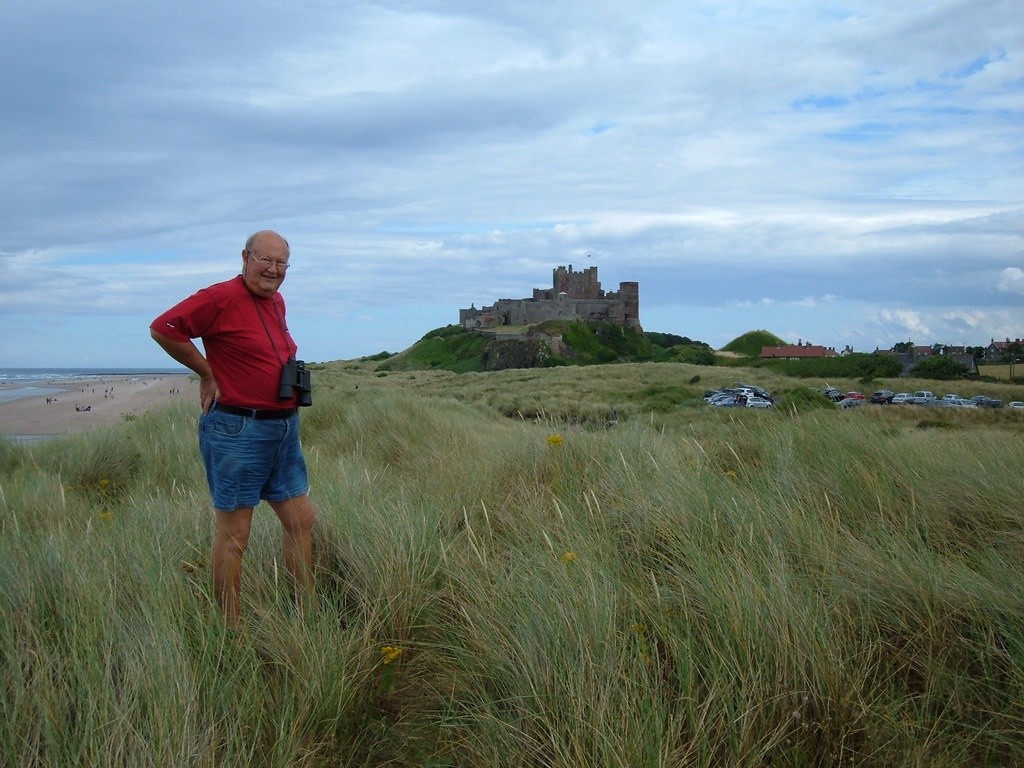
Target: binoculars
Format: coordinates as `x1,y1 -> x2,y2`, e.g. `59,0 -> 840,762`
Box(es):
278,359 -> 312,407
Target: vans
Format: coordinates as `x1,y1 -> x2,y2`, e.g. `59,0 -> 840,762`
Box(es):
913,391 -> 938,404
948,398 -> 977,409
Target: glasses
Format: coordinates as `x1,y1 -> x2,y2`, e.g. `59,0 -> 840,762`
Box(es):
251,255 -> 290,270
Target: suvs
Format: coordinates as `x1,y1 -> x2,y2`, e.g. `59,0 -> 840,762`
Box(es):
870,390 -> 895,405
978,399 -> 1004,408
942,394 -> 963,401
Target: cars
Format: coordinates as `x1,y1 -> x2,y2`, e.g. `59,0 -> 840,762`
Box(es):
1006,402 -> 1024,409
824,387 -> 864,401
704,384 -> 774,408
968,396 -> 987,406
836,398 -> 860,407
892,393 -> 915,406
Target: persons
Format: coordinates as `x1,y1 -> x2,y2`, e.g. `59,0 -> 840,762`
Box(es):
46,388 -> 179,413
146,231 -> 316,630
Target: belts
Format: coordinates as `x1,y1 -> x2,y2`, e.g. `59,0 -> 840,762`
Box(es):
210,399 -> 296,419
